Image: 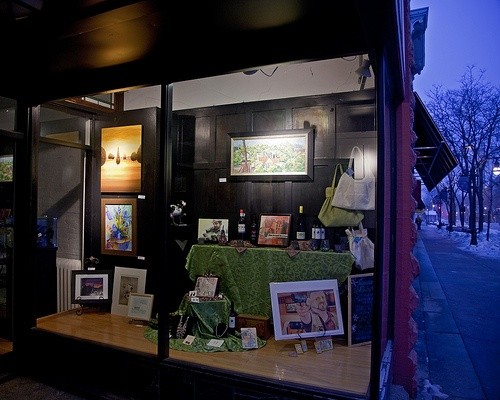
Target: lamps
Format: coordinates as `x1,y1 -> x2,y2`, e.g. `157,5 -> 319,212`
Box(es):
354,59 -> 371,77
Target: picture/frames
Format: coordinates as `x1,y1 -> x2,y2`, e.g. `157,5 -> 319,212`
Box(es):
128,293 -> 154,321
110,266 -> 147,316
71,270 -> 112,304
197,217 -> 231,244
256,213 -> 293,247
268,279 -> 344,340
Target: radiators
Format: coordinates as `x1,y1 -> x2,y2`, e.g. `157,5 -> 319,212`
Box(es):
56,258 -> 82,311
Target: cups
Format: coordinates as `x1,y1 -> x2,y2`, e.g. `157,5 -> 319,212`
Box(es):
320,239 -> 330,252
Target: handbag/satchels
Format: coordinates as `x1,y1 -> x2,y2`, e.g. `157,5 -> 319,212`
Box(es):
331,145 -> 375,211
317,163 -> 365,226
348,221 -> 374,270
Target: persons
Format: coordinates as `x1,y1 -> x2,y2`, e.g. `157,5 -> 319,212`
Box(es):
203,220 -> 223,242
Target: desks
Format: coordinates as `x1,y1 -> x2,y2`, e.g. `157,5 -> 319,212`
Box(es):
186,246 -> 356,325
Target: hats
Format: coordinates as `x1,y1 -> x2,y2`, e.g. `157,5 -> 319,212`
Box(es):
211,219 -> 222,223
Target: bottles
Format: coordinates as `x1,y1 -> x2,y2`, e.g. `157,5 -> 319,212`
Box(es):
229,302 -> 236,337
237,209 -> 246,240
251,213 -> 257,246
296,205 -> 307,241
2,254 -> 6,274
46,224 -> 54,246
312,219 -> 325,240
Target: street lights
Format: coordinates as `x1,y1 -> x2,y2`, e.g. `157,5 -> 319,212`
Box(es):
486,164 -> 500,244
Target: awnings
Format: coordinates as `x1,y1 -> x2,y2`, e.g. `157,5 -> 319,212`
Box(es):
412,90 -> 458,192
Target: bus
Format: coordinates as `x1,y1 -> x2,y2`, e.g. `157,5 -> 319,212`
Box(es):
424,210 -> 438,225
